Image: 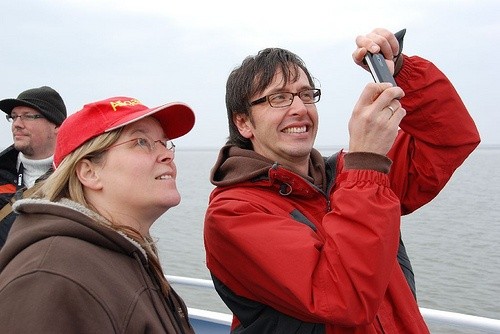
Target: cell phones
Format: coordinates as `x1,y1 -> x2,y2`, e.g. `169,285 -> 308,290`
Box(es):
364,51 -> 401,100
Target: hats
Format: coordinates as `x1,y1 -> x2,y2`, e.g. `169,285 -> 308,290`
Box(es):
54,97 -> 195,170
0,86 -> 67,126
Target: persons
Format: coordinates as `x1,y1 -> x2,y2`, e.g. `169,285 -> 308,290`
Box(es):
0,86 -> 67,248
203,29 -> 481,334
0,97 -> 196,334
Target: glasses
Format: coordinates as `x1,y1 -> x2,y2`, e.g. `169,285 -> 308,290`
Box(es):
88,134 -> 176,154
5,113 -> 47,122
248,88 -> 321,108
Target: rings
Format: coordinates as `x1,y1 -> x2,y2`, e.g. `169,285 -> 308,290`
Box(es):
387,106 -> 394,114
